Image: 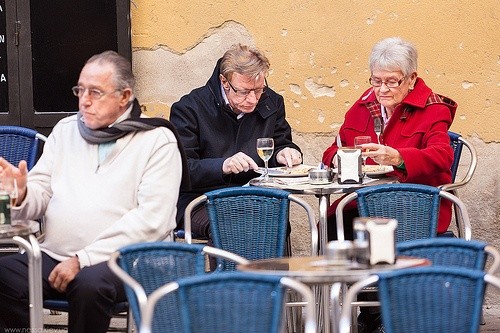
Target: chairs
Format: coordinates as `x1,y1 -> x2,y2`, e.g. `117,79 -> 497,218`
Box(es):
435,130 -> 487,326
335,182 -> 500,333
138,269 -> 319,333
0,125 -> 49,172
183,187 -> 323,332
107,241 -> 252,332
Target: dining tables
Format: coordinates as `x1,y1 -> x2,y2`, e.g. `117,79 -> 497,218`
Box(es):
0,217 -> 48,333
247,173 -> 402,256
234,253 -> 433,332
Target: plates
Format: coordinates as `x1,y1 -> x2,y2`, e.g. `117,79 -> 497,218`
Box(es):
332,164 -> 394,177
254,166 -> 316,177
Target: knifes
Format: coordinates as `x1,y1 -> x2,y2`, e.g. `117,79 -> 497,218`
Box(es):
249,166 -> 284,175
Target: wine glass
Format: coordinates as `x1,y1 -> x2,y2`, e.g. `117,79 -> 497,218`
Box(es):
256,138 -> 274,180
0,179 -> 17,209
354,136 -> 373,180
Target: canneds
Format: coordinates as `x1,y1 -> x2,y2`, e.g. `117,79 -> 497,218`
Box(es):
0,193 -> 11,229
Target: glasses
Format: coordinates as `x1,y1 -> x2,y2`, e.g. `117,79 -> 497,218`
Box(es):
72,86 -> 122,99
225,76 -> 268,97
369,72 -> 412,88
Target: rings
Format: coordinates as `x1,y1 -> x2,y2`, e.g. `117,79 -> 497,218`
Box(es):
374,156 -> 379,162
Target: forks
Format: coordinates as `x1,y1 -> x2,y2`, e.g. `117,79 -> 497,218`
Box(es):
373,118 -> 382,167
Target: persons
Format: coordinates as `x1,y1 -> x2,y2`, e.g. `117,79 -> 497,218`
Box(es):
0,50 -> 192,333
169,43 -> 303,274
313,37 -> 459,333
